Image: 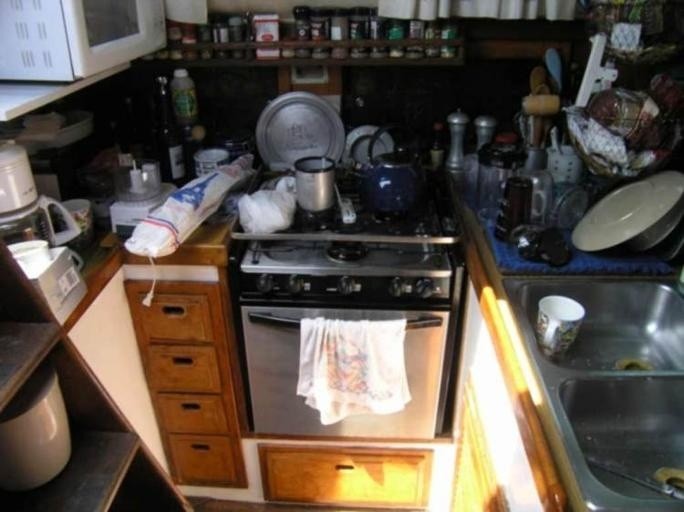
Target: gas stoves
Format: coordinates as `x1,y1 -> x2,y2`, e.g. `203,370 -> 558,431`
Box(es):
234,161 -> 461,304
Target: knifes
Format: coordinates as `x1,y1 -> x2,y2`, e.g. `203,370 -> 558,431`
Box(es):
583,454 -> 683,503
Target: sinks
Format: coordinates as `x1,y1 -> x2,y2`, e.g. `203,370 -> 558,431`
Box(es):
515,280 -> 684,372
557,373 -> 684,502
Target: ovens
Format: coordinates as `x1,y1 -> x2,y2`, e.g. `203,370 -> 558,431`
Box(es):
234,300 -> 458,441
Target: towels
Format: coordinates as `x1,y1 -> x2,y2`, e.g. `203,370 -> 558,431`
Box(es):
295,315 -> 410,425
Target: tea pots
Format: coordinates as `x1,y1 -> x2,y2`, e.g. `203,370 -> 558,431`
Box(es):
353,121 -> 424,216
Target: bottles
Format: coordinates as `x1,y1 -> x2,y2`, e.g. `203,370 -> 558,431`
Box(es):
436,115 -> 588,242
152,67 -> 202,188
163,6 -> 455,59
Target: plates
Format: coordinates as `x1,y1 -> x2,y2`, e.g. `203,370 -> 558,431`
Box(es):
346,126 -> 395,164
255,91 -> 344,172
18,106 -> 96,153
572,169 -> 684,262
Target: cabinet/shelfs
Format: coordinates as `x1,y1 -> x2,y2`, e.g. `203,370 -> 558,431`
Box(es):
258,438 -> 441,512
443,374 -> 513,512
0,247 -> 194,512
121,247 -> 250,512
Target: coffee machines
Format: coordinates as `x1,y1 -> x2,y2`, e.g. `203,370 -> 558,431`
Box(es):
0,138 -> 81,251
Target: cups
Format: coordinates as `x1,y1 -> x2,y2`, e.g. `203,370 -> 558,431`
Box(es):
0,365 -> 71,493
5,242 -> 51,276
193,148 -> 229,178
51,198 -> 93,251
538,294 -> 586,362
293,156 -> 334,210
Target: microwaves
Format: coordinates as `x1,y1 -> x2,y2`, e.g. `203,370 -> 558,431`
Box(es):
0,1 -> 166,84
